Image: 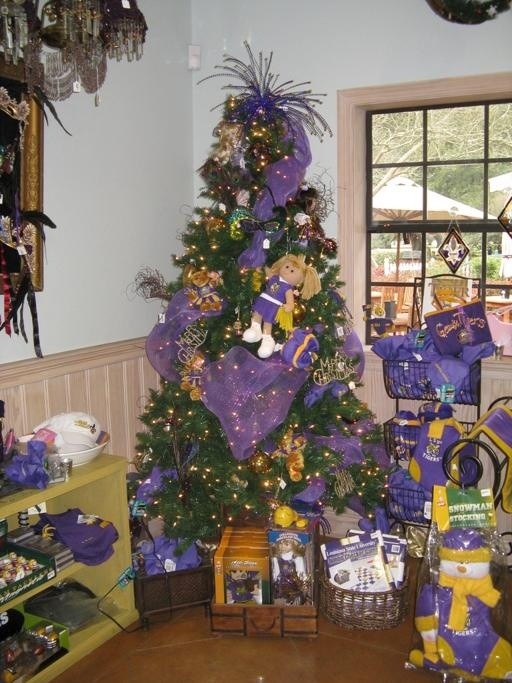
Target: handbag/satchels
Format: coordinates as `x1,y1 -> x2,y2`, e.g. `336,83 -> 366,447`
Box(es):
408,424 -> 467,493
34,507 -> 118,566
423,297 -> 492,356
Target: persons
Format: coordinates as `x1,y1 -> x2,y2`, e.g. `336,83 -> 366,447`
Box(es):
271,531 -> 309,607
242,253 -> 321,357
0,550 -> 40,594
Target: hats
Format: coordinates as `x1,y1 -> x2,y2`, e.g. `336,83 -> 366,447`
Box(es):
59,409 -> 101,451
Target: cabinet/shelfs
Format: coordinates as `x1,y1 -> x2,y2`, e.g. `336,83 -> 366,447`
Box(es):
381,273 -> 483,533
0,437 -> 140,683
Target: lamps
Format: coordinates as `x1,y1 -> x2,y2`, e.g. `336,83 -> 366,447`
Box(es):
0,0 -> 147,106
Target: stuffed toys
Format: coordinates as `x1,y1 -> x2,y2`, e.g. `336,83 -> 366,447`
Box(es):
180,351 -> 205,401
185,266 -> 224,313
408,521 -> 512,682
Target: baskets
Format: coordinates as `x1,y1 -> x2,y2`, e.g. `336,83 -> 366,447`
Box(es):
383,485 -> 434,529
316,561 -> 414,632
383,415 -> 480,461
383,359 -> 482,406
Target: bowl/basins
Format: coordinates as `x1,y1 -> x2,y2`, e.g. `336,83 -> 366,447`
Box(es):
13,431 -> 112,467
31,412 -> 100,454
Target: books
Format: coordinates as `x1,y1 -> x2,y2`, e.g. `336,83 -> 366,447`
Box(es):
320,524 -> 408,619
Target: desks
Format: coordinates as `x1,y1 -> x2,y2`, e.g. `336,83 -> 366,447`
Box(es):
368,290 -> 511,355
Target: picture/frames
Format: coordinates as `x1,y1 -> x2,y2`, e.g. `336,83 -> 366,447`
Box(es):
0,63 -> 45,293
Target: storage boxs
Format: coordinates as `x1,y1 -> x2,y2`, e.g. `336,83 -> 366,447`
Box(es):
211,577 -> 319,639
